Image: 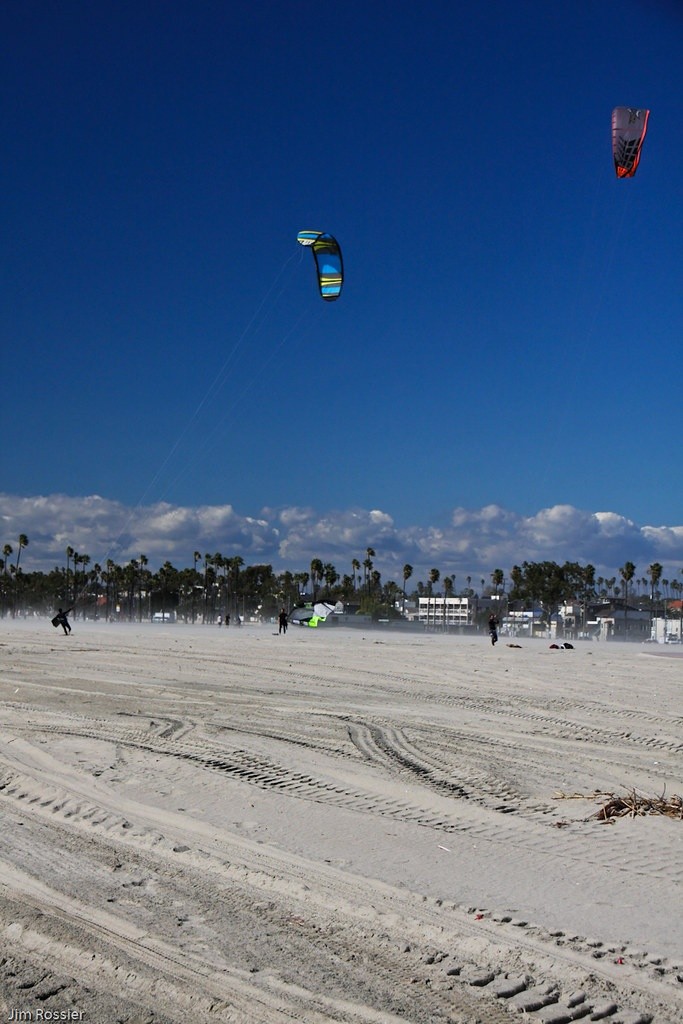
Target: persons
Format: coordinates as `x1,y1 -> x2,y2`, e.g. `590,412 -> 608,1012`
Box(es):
278,608 -> 289,634
488,614 -> 499,646
54,608 -> 72,636
217,614 -> 241,627
